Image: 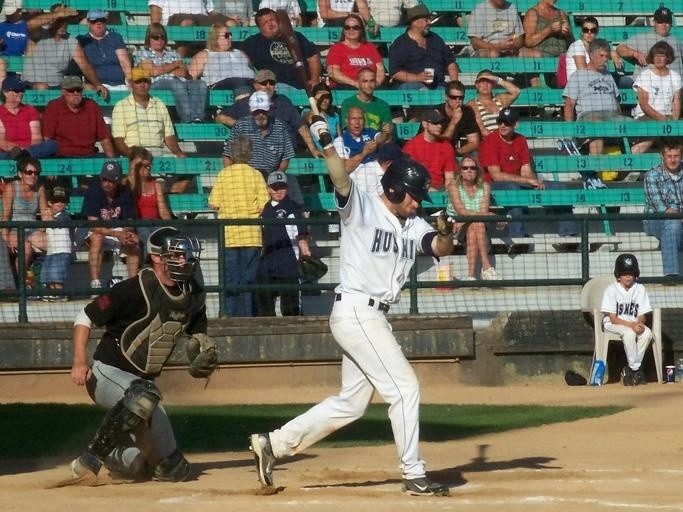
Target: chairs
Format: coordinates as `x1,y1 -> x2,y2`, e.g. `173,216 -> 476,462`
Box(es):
581,277 -> 664,383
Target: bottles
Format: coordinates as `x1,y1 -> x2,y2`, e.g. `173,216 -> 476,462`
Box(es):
676,358 -> 682,383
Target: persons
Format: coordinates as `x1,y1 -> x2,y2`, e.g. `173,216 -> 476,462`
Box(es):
250,113 -> 457,497
71,229 -> 217,486
600,254 -> 652,387
0,2 -> 683,318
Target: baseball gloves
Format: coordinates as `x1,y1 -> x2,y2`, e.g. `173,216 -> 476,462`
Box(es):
186,333 -> 219,378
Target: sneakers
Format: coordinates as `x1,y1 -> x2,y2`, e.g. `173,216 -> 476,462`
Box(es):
622,365 -> 645,386
75,448 -> 101,481
251,431 -> 273,488
90,276 -> 102,298
402,476 -> 449,497
465,268 -> 503,290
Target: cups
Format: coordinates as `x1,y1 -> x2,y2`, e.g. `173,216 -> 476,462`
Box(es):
664,365 -> 676,385
423,66 -> 435,85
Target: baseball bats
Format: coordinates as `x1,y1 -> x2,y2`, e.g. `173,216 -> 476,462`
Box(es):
275,9 -> 331,147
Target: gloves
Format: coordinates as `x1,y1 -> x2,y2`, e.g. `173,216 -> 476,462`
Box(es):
308,113 -> 333,151
429,209 -> 455,238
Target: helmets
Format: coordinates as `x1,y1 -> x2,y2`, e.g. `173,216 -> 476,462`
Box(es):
613,253 -> 640,277
147,225 -> 202,284
380,156 -> 433,205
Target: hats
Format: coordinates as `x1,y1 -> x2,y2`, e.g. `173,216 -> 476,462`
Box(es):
266,171 -> 287,188
98,160 -> 124,180
407,4 -> 433,24
1,0 -> 151,92
47,186 -> 70,206
254,70 -> 275,85
248,90 -> 270,115
497,107 -> 518,121
655,7 -> 672,24
420,108 -> 446,124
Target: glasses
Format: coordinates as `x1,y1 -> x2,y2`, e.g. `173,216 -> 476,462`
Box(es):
342,24 -> 361,32
220,32 -> 235,38
462,166 -> 477,171
582,26 -> 597,33
448,94 -> 464,99
24,169 -> 40,176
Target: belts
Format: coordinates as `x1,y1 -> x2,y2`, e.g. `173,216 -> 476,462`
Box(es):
333,291 -> 390,313
